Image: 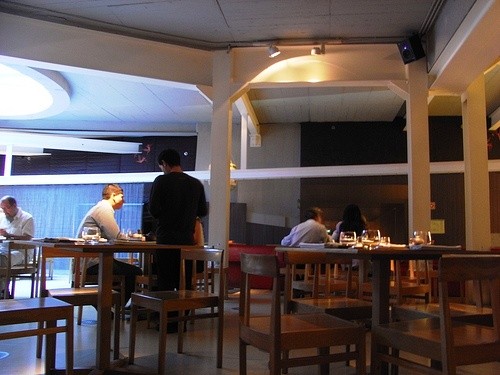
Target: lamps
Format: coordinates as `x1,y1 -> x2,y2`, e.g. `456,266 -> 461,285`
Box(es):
267,39 -> 280,58
311,39 -> 326,56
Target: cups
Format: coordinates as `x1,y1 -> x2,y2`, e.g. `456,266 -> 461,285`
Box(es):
409,238 -> 423,250
339,232 -> 357,246
357,237 -> 362,247
381,237 -> 390,246
361,229 -> 381,249
413,230 -> 431,247
83,227 -> 100,244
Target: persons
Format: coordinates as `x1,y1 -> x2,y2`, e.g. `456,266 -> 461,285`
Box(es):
279,207 -> 329,298
72,183 -> 143,320
0,196 -> 35,300
331,203 -> 375,277
148,147 -> 208,335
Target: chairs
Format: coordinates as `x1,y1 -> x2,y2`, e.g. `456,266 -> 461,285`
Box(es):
0,240 -> 500,375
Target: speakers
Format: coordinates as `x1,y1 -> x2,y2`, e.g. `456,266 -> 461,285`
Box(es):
396,36 -> 426,65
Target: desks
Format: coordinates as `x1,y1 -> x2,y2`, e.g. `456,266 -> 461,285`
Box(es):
14,240 -> 203,375
274,245 -> 463,375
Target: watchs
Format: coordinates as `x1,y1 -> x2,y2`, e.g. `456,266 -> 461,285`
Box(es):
10,236 -> 15,240
